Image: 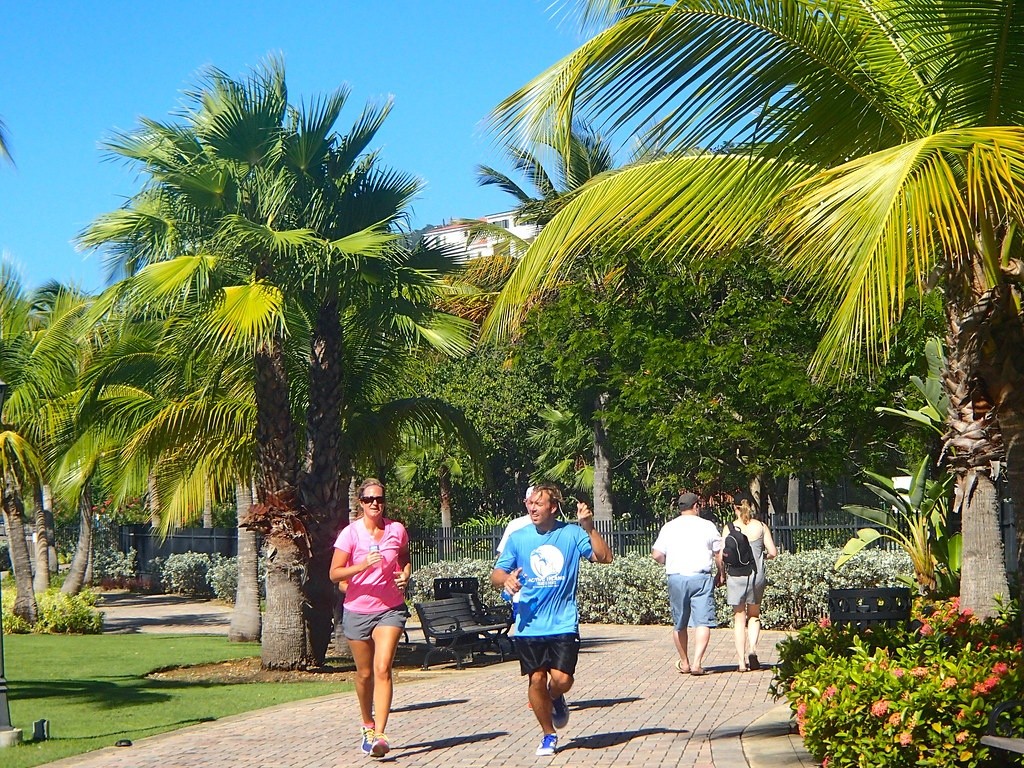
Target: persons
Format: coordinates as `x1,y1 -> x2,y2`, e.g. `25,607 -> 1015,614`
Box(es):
652,492 -> 722,675
714,493 -> 777,672
328,478 -> 411,757
496,486 -> 534,711
491,485 -> 613,756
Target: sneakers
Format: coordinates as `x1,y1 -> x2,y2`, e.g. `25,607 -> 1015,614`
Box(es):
535,733 -> 558,756
552,694 -> 569,729
360,720 -> 375,754
369,733 -> 390,757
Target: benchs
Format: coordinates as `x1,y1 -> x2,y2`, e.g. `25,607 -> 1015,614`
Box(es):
435,576 -> 516,652
413,596 -> 507,670
980,685 -> 1024,768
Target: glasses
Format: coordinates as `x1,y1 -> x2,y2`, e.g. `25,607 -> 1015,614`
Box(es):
558,496 -> 581,524
360,496 -> 385,504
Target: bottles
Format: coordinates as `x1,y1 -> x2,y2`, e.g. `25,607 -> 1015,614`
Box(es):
369,536 -> 382,568
501,574 -> 527,601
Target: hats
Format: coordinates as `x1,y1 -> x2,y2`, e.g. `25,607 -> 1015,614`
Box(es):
734,493 -> 752,508
526,486 -> 534,499
678,492 -> 705,511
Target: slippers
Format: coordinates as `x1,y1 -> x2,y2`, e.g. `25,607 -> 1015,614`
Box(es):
675,659 -> 691,673
691,668 -> 705,676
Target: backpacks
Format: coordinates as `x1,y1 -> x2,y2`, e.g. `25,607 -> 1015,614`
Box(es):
722,521 -> 758,577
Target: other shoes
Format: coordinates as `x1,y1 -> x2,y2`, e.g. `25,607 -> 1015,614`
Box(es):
749,654 -> 760,670
527,700 -> 534,711
738,668 -> 747,672
